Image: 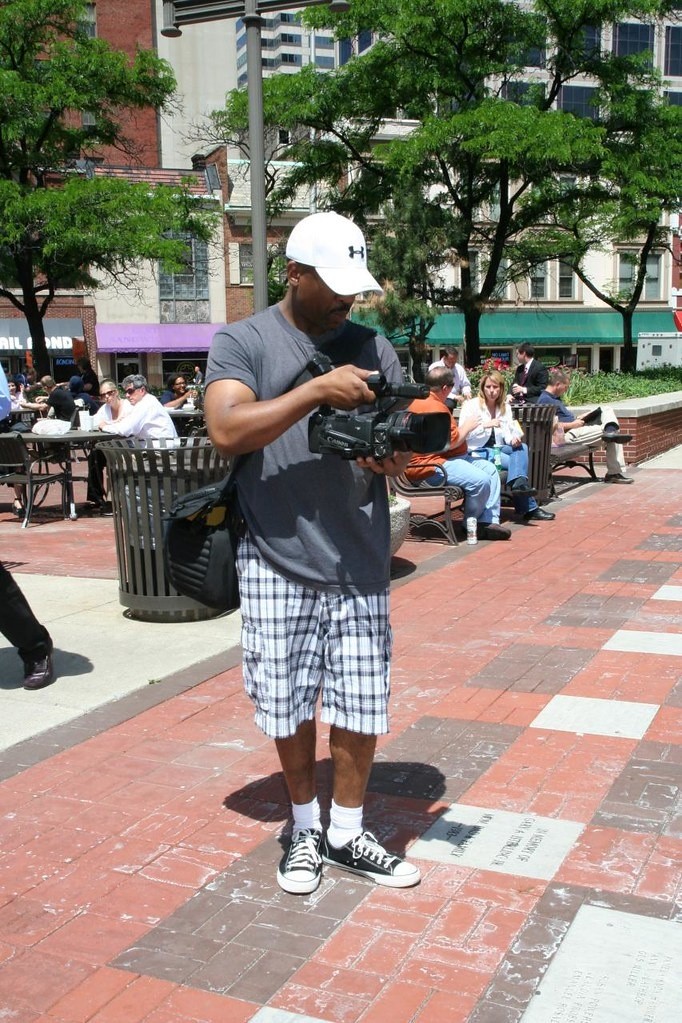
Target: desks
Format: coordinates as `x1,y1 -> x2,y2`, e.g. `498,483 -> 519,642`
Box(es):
21,432 -> 120,520
9,409 -> 37,433
169,409 -> 204,437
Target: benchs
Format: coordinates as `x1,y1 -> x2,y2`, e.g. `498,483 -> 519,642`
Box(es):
384,447 -> 510,547
546,444 -> 599,499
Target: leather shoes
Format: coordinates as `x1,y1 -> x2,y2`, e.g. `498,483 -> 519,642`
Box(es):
601,431 -> 632,443
605,474 -> 634,483
511,484 -> 538,496
21,634 -> 53,689
523,507 -> 555,520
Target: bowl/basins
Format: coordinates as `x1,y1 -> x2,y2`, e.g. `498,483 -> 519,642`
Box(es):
182,406 -> 196,411
172,410 -> 184,414
164,407 -> 174,413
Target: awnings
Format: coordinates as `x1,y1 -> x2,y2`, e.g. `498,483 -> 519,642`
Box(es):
348,304 -> 422,347
409,310 -> 682,345
0,317 -> 83,357
96,323 -> 228,354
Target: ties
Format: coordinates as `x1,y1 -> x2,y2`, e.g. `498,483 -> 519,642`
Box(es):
519,368 -> 527,403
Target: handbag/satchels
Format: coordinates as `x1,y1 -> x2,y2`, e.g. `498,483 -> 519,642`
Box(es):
161,475 -> 241,609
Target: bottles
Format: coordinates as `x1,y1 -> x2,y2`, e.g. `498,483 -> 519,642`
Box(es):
493,444 -> 502,471
467,516 -> 478,545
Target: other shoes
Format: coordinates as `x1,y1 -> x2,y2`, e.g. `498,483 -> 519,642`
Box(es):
102,507 -> 117,515
78,504 -> 101,512
479,522 -> 511,540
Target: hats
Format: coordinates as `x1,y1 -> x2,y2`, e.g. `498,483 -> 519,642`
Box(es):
285,211 -> 384,295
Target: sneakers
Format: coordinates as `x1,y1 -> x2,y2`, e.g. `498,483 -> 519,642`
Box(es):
320,828 -> 422,888
277,828 -> 322,893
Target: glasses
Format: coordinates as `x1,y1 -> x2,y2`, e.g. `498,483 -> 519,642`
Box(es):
98,390 -> 116,399
122,388 -> 137,395
447,383 -> 454,388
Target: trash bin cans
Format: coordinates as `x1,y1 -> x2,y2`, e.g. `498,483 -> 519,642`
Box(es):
511,404 -> 556,499
95,436 -> 235,621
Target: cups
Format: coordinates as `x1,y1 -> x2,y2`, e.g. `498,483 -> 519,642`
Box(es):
187,397 -> 194,411
79,409 -> 90,430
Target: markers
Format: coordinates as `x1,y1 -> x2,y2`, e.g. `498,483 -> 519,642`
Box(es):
89,430 -> 99,432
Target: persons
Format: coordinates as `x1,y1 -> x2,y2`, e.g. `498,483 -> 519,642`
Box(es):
0,354 -> 54,691
0,420 -> 26,517
458,369 -> 556,520
98,375 -> 181,515
398,365 -> 513,541
537,370 -> 634,484
15,365 -> 44,392
7,379 -> 34,423
65,375 -> 100,415
202,212 -> 424,894
21,375 -> 75,421
427,347 -> 472,413
87,377 -> 133,507
194,366 -> 202,385
160,374 -> 198,434
506,343 -> 549,404
68,355 -> 99,397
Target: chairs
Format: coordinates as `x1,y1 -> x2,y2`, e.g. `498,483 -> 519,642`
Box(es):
0,433 -> 67,529
36,404 -> 93,464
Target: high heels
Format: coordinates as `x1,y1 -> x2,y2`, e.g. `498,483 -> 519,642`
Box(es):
12,498 -> 26,517
